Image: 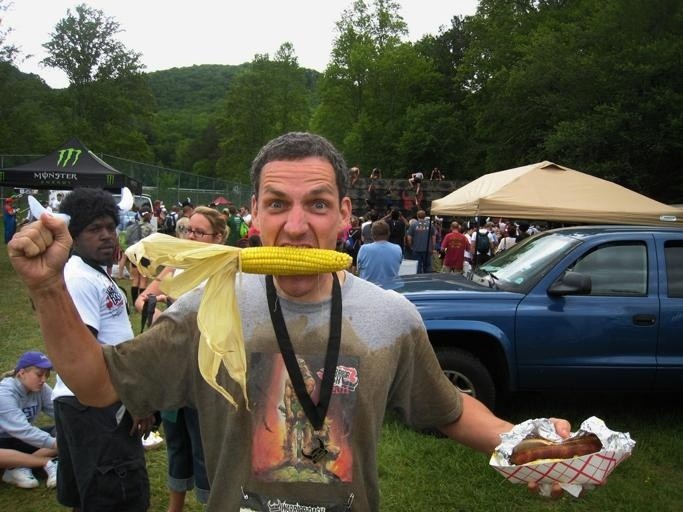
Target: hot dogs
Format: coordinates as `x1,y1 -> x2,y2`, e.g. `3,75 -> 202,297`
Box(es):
509,435 -> 602,465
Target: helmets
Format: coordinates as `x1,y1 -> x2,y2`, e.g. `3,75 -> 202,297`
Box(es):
6,198 -> 12,202
11,195 -> 18,199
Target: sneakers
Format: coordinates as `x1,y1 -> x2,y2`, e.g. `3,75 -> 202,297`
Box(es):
2,467 -> 39,489
43,458 -> 59,489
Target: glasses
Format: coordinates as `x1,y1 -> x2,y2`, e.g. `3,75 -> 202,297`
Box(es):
187,229 -> 214,238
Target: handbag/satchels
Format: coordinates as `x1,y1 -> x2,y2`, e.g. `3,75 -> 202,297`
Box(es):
240,222 -> 249,238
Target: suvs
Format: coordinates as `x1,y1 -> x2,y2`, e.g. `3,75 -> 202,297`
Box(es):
373,225 -> 682,436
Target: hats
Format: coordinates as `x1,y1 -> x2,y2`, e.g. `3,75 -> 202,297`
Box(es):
15,351 -> 53,373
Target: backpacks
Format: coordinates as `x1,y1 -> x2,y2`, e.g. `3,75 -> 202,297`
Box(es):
164,213 -> 175,233
126,224 -> 141,245
476,231 -> 490,253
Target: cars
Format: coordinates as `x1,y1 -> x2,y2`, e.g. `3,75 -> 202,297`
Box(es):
111,193 -> 158,232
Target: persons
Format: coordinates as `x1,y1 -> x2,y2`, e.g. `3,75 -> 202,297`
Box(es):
1,346 -> 58,491
136,205 -> 228,512
5,130 -> 608,510
49,184 -> 150,512
0,161 -> 568,309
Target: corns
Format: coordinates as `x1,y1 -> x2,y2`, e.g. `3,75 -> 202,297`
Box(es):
237,246 -> 354,278
